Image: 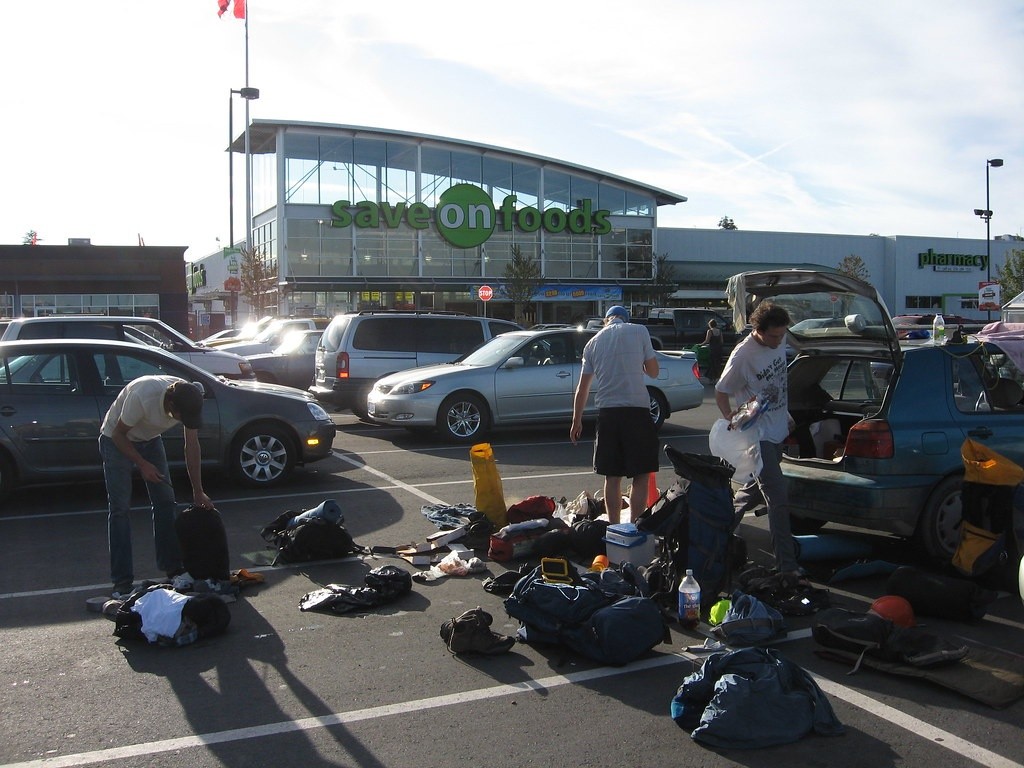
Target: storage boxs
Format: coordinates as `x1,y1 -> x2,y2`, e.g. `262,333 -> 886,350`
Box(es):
602,523 -> 656,566
487,532 -> 541,562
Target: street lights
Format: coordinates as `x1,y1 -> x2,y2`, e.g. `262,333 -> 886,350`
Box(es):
229,87 -> 259,330
974,159 -> 1004,323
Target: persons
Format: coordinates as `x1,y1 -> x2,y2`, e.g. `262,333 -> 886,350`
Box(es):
714,300 -> 811,589
571,306 -> 660,526
99,375 -> 215,599
699,319 -> 723,386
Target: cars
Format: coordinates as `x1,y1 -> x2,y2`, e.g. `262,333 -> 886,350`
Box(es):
0,339 -> 337,487
368,329 -> 704,445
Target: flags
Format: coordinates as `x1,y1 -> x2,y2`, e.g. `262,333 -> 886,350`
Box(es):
218,0 -> 245,23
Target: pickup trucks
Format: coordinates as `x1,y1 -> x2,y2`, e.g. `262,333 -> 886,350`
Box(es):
586,309 -> 754,380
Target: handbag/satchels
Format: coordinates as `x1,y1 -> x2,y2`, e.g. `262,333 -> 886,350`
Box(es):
469,495 -> 668,668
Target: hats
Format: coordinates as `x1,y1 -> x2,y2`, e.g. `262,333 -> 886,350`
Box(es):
173,382 -> 203,429
606,306 -> 628,322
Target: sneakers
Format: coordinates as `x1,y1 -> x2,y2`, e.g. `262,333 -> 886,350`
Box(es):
440,606 -> 516,655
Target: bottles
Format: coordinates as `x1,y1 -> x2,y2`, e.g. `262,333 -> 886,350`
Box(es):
933,313 -> 944,344
678,569 -> 700,628
592,555 -> 608,573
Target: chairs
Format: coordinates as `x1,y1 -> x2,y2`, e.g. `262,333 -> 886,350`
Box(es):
544,340 -> 565,364
525,343 -> 543,364
954,395 -> 975,412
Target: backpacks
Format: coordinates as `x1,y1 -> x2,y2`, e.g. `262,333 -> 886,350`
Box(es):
259,509 -> 349,562
176,502 -> 231,581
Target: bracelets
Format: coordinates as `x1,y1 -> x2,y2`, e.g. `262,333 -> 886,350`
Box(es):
134,458 -> 146,471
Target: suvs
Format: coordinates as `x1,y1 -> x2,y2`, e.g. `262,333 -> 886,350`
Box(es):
0,304 -> 581,427
721,270 -> 1024,557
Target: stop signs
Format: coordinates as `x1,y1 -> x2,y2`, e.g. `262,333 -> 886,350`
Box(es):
478,286 -> 493,301
